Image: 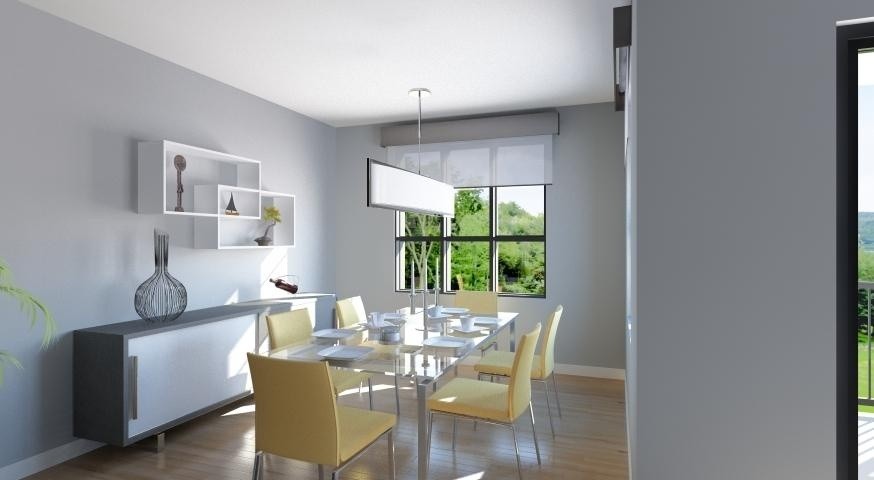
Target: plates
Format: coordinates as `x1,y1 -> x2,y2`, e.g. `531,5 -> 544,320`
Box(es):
421,336 -> 471,348
318,344 -> 375,361
418,347 -> 471,358
446,331 -> 488,338
473,317 -> 501,324
311,327 -> 357,339
447,325 -> 490,333
441,308 -> 470,314
358,321 -> 396,330
381,313 -> 405,320
426,314 -> 452,319
427,317 -> 452,323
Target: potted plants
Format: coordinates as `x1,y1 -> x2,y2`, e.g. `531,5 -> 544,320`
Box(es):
252,203 -> 283,247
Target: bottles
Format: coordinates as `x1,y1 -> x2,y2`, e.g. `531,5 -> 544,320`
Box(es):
268,278 -> 298,294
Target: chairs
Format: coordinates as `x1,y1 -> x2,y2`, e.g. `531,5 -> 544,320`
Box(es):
454,289 -> 501,380
264,307 -> 376,411
244,350 -> 396,479
471,302 -> 567,442
425,321 -> 544,478
333,295 -> 405,411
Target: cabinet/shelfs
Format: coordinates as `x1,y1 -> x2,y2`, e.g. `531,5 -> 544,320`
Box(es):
71,289 -> 337,455
132,135 -> 298,252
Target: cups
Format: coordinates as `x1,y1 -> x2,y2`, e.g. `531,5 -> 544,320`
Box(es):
460,315 -> 474,330
430,323 -> 442,332
428,307 -> 442,317
369,312 -> 384,327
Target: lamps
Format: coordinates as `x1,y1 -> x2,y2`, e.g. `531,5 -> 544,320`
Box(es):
362,86 -> 457,219
